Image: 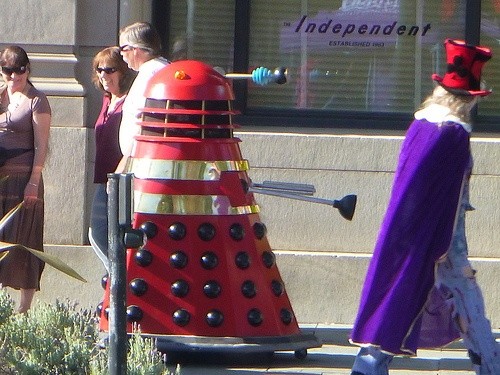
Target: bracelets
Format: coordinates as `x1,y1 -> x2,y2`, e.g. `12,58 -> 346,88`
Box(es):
28,182 -> 38,188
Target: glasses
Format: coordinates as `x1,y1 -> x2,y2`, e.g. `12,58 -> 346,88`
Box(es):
119,44 -> 128,51
97,67 -> 117,74
2,64 -> 28,75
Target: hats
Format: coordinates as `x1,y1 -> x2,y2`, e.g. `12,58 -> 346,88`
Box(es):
432,38 -> 493,97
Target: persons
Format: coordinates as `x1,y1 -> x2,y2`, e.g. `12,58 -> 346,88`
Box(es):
118,22 -> 174,156
91,47 -> 139,257
349,38 -> 500,375
0,46 -> 51,315
